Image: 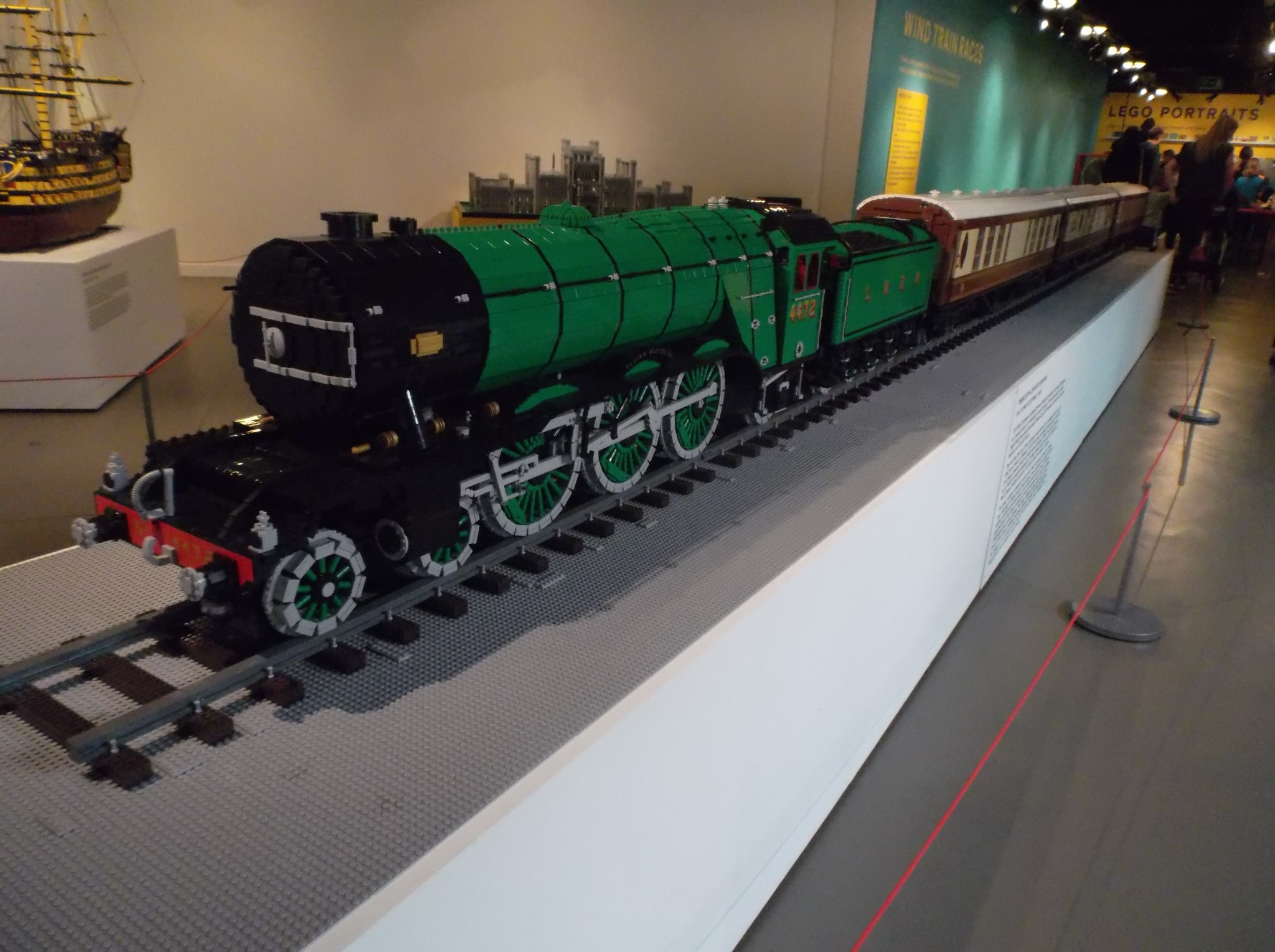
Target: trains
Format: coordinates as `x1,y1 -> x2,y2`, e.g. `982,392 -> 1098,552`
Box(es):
66,181 -> 1150,639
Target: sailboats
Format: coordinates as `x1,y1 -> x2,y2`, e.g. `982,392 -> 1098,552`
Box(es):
0,0 -> 146,254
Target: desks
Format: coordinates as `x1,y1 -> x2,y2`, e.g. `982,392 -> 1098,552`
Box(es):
0,228 -> 187,409
0,251 -> 1176,951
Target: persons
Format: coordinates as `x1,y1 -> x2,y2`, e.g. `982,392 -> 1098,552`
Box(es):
1102,117 -> 1193,252
1227,145 -> 1275,261
1168,115 -> 1239,296
